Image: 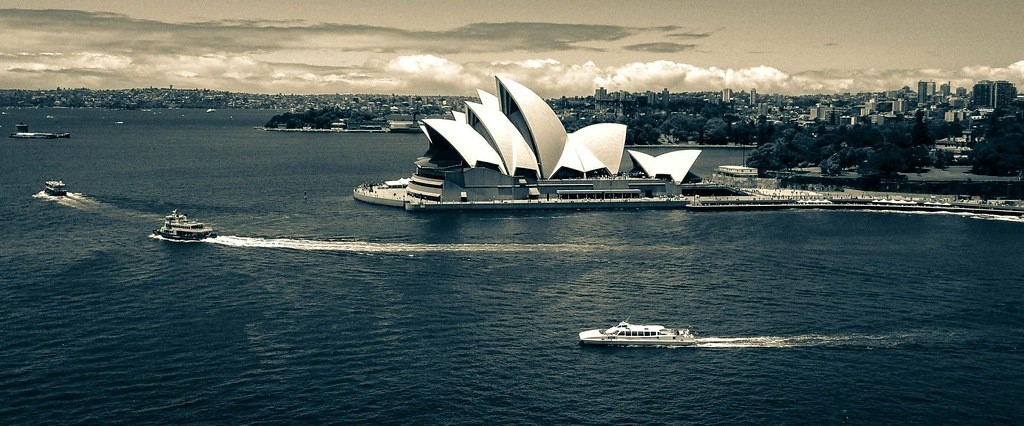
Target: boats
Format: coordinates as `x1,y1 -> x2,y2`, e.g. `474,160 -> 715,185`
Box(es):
152,209 -> 214,241
45,178 -> 66,196
578,321 -> 695,346
8,131 -> 57,139
718,164 -> 759,177
206,108 -> 216,113
